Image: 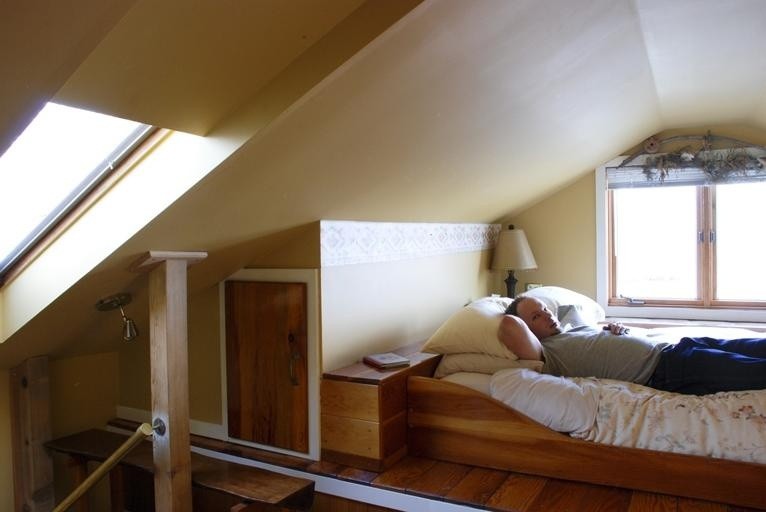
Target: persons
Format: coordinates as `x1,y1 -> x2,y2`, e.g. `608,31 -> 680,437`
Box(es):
498,298 -> 766,396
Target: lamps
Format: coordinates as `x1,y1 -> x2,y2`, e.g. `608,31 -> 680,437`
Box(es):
487,220 -> 538,299
96,292 -> 140,341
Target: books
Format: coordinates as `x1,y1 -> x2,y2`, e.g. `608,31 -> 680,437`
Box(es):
363,352 -> 410,369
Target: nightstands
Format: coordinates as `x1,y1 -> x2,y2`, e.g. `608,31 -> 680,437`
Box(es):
322,340 -> 442,475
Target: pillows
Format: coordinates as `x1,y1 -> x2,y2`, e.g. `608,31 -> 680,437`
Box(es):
420,295 -> 520,361
520,285 -> 606,325
429,348 -> 543,378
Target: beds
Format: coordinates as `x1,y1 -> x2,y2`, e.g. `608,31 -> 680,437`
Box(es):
412,284 -> 766,512
